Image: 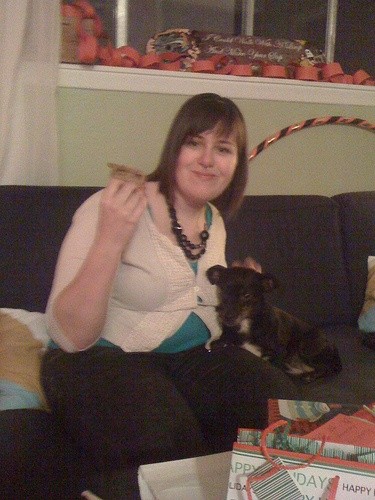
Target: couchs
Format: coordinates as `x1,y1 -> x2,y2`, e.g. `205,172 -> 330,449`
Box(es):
0,184 -> 375,403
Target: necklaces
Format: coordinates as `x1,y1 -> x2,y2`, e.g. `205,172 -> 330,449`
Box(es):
165,190 -> 212,259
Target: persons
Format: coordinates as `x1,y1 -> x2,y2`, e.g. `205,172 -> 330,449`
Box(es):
42,94 -> 300,500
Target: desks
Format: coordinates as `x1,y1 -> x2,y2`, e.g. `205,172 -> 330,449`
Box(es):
138,452 -> 246,499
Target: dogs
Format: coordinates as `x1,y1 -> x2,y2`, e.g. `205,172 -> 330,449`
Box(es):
204,264 -> 342,386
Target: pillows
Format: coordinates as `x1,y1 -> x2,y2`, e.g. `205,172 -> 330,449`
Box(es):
0,307 -> 65,408
